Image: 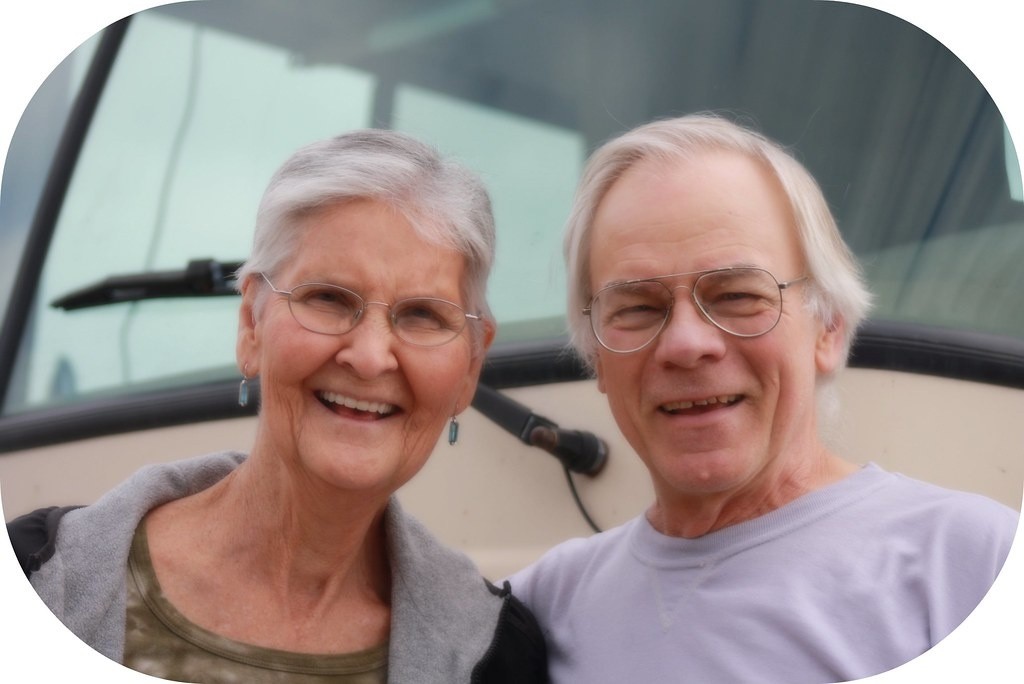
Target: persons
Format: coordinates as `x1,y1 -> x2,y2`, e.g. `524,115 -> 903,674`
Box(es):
493,111 -> 1020,684
4,129 -> 549,684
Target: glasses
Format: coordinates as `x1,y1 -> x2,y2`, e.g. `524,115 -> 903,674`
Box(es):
579,266 -> 811,354
260,272 -> 487,347
